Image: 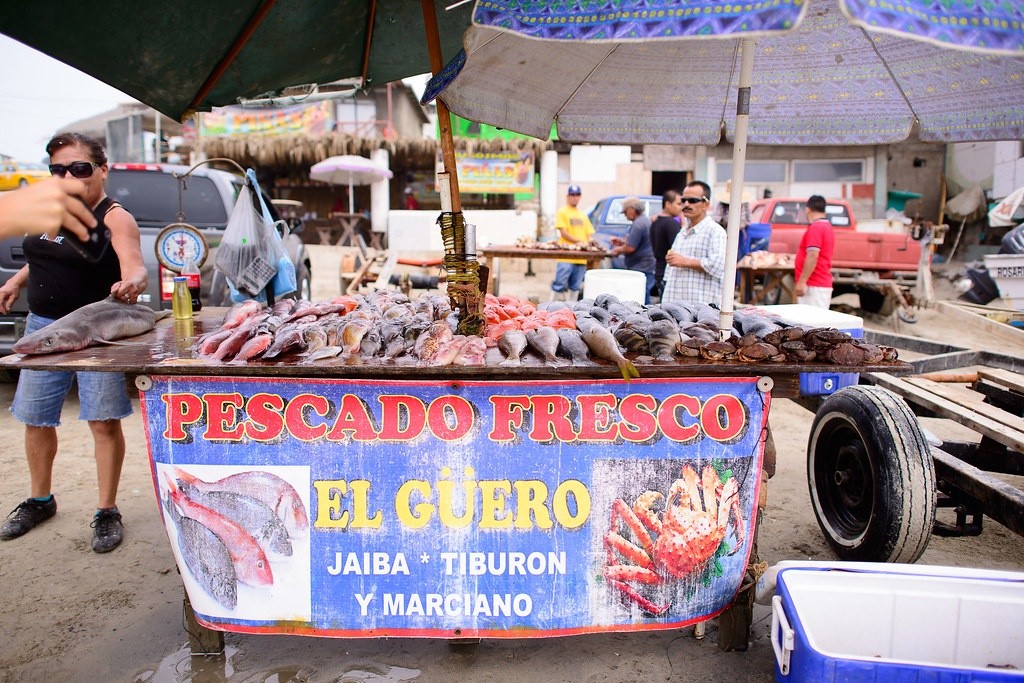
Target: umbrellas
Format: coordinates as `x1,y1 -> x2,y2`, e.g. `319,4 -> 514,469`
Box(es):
988,188 -> 1024,227
945,185 -> 985,262
0,0 -> 1024,345
312,155 -> 392,245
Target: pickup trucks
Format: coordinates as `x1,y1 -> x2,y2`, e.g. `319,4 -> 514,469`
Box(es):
589,196 -> 663,296
741,198 -> 935,316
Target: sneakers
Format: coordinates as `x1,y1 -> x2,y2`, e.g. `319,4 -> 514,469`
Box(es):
91,508 -> 122,553
0,494 -> 56,541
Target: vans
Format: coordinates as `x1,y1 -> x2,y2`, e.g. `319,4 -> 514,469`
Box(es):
106,162 -> 312,312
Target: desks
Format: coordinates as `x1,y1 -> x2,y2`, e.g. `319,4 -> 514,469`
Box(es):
477,244 -> 619,298
739,267 -> 797,304
0,306 -> 918,653
332,212 -> 367,247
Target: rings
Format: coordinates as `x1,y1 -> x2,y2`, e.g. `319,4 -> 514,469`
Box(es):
125,293 -> 130,299
127,299 -> 131,303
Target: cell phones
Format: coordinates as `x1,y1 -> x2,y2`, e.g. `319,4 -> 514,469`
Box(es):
58,222 -> 112,263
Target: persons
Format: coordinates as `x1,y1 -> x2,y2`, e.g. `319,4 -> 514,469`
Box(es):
0,132 -> 149,550
662,180 -> 726,308
552,185 -> 596,302
795,195 -> 835,310
0,176 -> 96,240
153,131 -> 169,163
651,191 -> 687,300
405,187 -> 419,209
611,198 -> 655,305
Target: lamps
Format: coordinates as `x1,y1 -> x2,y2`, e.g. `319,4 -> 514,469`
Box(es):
914,157 -> 926,169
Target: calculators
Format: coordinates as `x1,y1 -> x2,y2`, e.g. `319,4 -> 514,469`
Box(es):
237,257 -> 277,295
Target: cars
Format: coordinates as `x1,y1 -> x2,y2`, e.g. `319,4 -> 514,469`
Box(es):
0,161 -> 53,383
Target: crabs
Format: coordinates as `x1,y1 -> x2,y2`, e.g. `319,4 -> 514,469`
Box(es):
677,324 -> 901,364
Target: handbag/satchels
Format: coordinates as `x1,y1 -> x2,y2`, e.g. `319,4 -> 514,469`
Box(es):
213,168 -> 298,303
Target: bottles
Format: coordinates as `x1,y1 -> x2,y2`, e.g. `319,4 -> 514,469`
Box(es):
171,277 -> 192,320
181,254 -> 202,311
175,320 -> 194,359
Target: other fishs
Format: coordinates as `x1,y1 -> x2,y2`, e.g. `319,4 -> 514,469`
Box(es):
12,293 -> 174,356
201,282 -> 787,382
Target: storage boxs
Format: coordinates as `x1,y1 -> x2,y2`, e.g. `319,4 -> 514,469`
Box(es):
764,299 -> 865,396
752,558 -> 1023,683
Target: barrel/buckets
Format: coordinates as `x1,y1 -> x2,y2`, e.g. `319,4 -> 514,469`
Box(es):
584,269 -> 646,306
746,222 -> 771,253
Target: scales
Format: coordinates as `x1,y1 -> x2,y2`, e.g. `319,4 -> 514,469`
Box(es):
157,157 -> 248,273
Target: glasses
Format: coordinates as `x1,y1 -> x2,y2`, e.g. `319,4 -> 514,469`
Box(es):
681,198 -> 704,204
49,160 -> 98,178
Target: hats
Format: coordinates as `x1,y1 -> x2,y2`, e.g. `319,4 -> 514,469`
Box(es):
567,185 -> 581,195
619,196 -> 646,212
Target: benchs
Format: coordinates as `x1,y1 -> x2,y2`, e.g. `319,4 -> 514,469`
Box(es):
315,226 -> 337,245
370,230 -> 385,252
